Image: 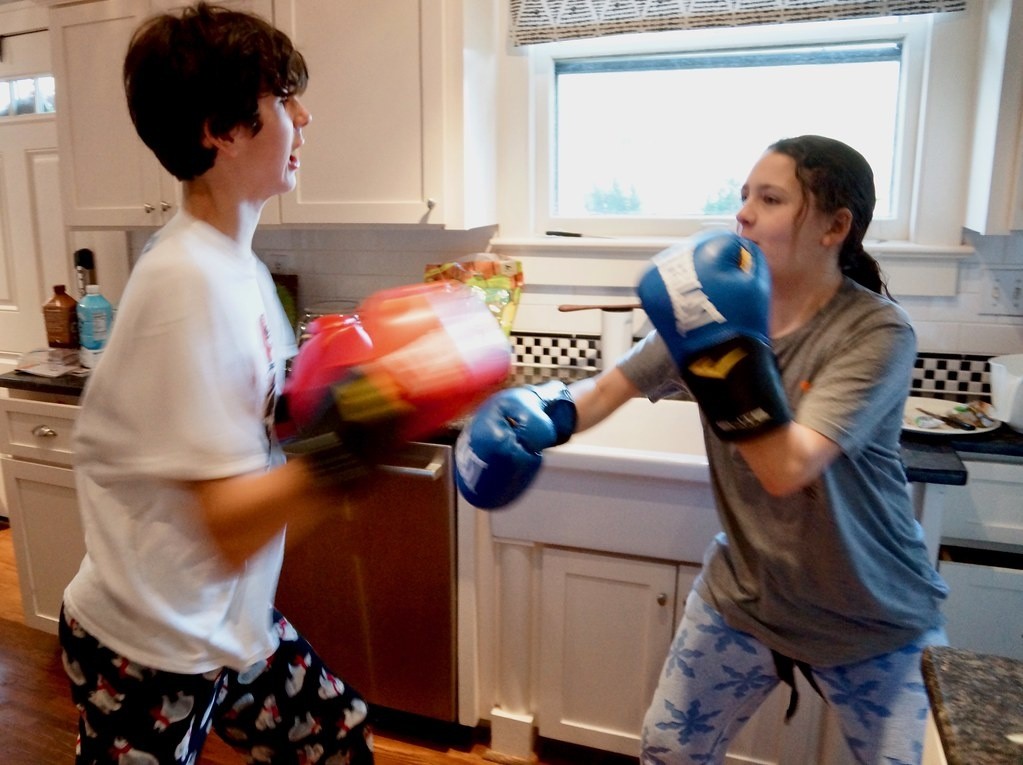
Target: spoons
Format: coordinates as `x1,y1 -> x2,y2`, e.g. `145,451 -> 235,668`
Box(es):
969,406 -> 994,428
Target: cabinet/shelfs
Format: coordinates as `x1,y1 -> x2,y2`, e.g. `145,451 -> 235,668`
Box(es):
0,389 -> 84,637
458,471 -> 946,765
46,0 -> 498,232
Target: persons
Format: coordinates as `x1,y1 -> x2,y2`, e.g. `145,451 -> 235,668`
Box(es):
450,135 -> 949,765
58,1 -> 510,765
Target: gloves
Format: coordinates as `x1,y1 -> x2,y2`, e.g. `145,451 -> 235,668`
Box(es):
452,381 -> 581,509
633,228 -> 792,442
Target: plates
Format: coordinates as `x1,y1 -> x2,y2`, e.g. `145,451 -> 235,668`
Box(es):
901,396 -> 1002,435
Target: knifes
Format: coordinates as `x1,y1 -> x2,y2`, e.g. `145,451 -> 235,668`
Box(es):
915,407 -> 976,431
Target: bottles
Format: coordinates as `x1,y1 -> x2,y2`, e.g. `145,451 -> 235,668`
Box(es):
76,284 -> 113,368
43,284 -> 81,365
